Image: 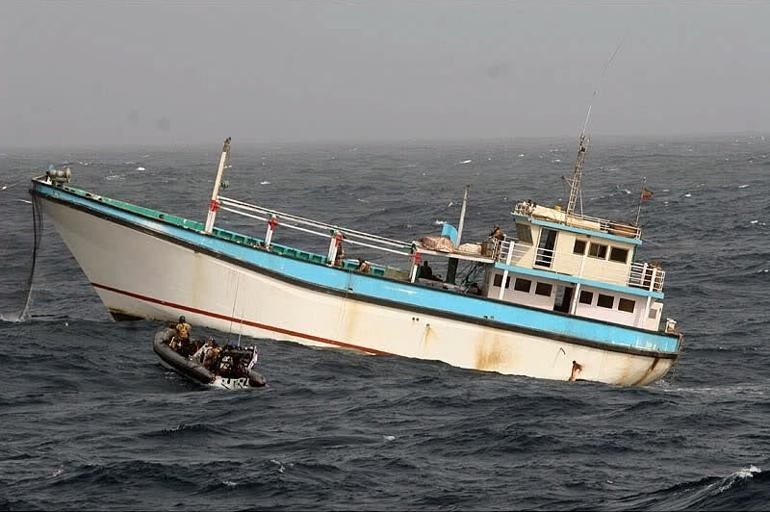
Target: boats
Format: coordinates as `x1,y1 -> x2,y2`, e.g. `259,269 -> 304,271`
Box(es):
29,88 -> 684,389
151,323 -> 267,390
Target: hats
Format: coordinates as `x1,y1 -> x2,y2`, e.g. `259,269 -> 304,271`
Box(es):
179,316 -> 185,322
212,340 -> 219,345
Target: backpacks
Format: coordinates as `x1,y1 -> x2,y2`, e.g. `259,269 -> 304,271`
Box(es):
178,324 -> 188,340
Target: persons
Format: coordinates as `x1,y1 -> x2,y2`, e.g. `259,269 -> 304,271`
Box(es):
488,224 -> 504,246
355,256 -> 370,273
419,260 -> 432,279
175,314 -> 193,346
334,241 -> 346,268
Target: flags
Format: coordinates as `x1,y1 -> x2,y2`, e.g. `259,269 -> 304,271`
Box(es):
640,187 -> 655,201
442,223 -> 458,246
